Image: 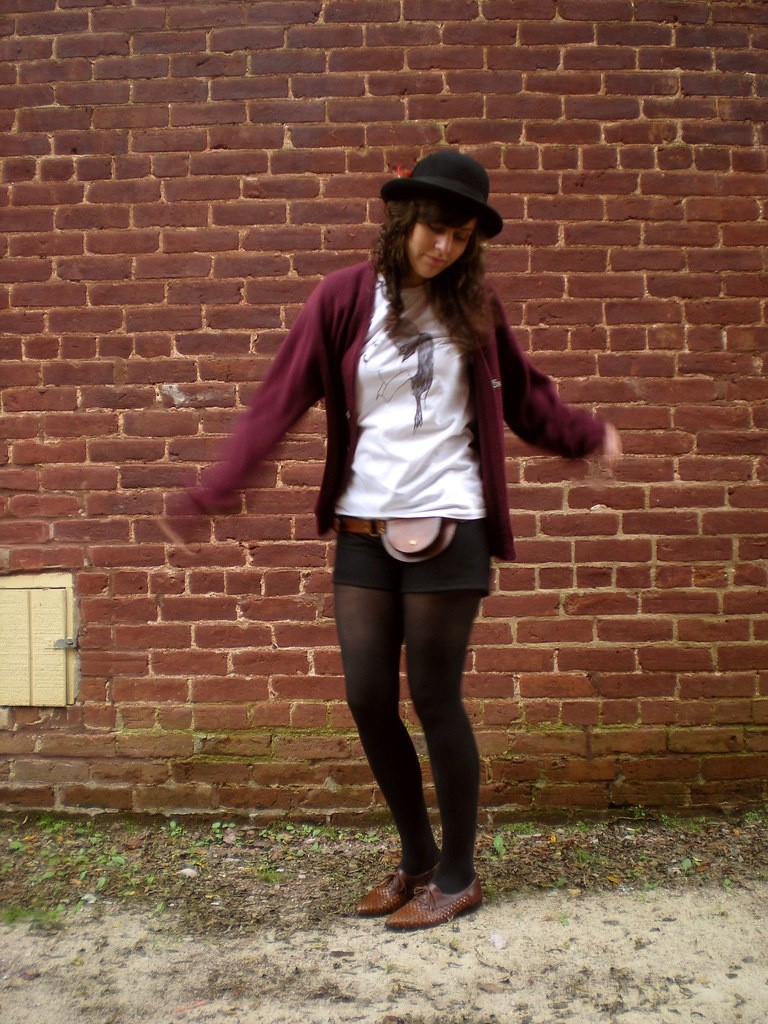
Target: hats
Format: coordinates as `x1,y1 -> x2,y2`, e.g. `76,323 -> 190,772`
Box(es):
380,150 -> 504,240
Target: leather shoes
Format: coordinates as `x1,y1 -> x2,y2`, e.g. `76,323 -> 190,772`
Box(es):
355,859 -> 442,917
385,873 -> 483,928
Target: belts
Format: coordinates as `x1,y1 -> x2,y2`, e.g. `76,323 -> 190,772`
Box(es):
334,517 -> 457,562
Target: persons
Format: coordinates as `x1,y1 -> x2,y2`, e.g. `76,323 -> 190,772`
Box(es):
157,149 -> 624,930
